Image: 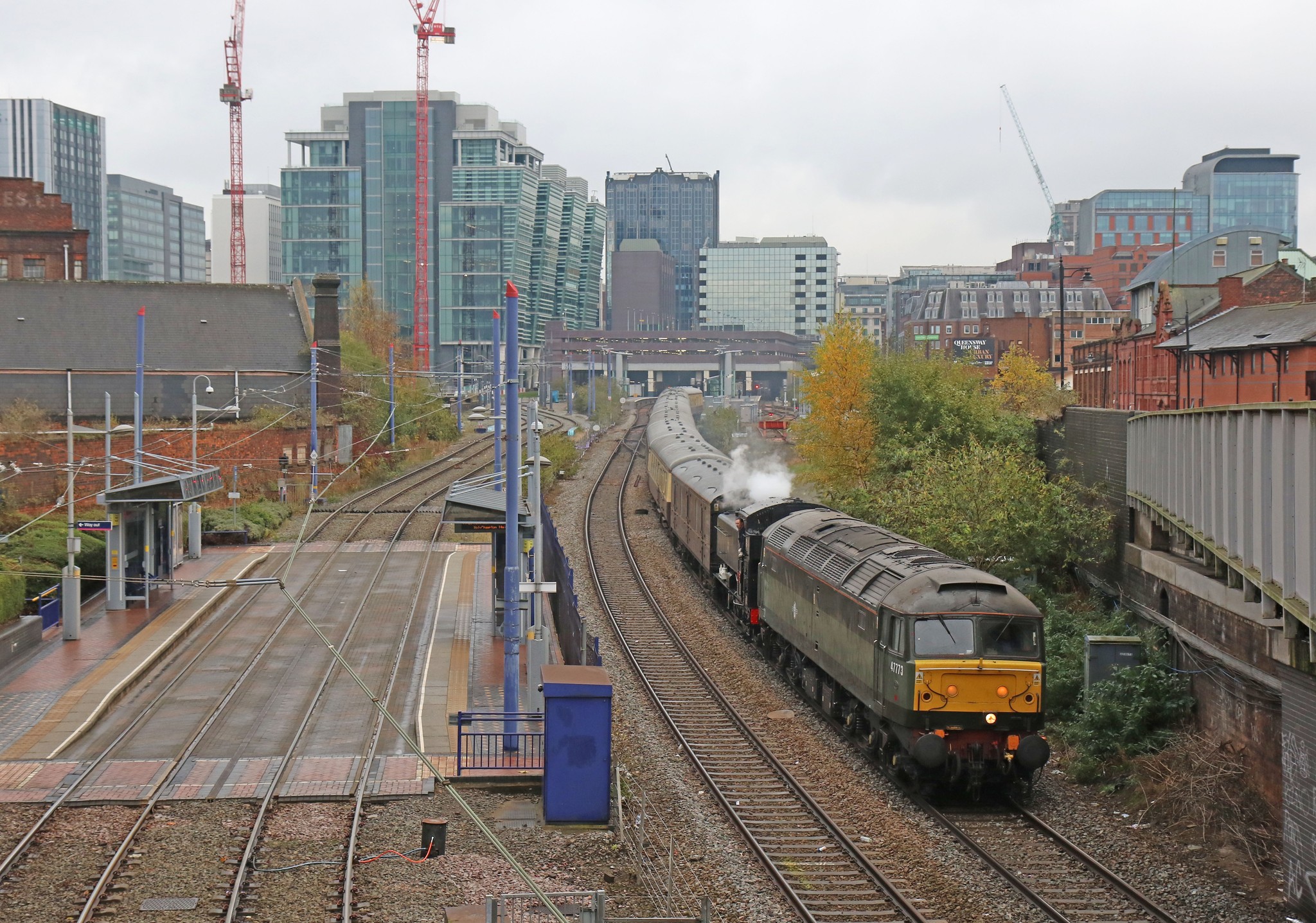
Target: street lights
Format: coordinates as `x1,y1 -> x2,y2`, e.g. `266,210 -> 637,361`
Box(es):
1058,257 -> 1095,389
715,345 -> 729,396
1086,348 -> 1107,409
1162,310 -> 1190,409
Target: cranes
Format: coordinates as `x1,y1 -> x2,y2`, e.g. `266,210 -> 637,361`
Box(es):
998,83 -> 1064,242
219,0 -> 254,283
410,0 -> 458,382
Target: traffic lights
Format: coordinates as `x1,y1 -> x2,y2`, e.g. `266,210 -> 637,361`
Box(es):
795,407 -> 798,409
755,385 -> 759,389
769,413 -> 773,416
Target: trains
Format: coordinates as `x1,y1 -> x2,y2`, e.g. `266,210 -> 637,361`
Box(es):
645,386 -> 1047,804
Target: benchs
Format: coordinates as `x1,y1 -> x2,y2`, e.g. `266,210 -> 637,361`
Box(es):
126,571 -> 159,607
